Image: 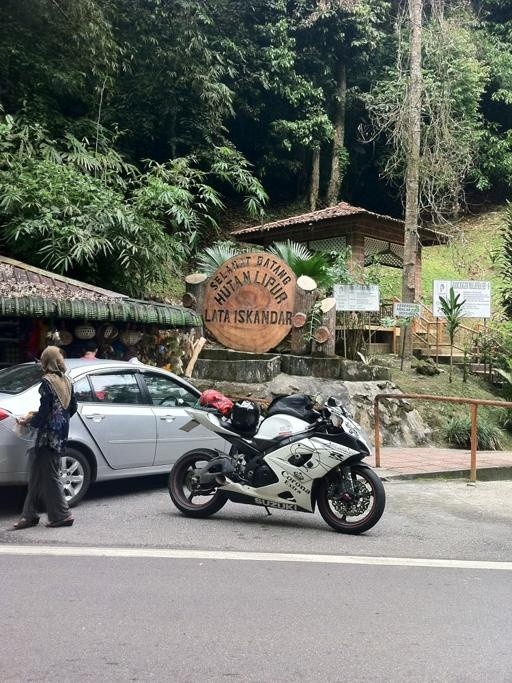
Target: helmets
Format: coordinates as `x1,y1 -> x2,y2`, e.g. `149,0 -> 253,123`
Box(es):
231,398 -> 259,432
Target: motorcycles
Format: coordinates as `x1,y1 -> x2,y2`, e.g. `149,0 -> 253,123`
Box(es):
167,393 -> 386,534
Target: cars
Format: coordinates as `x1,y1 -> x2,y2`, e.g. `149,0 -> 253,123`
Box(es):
0,356 -> 264,509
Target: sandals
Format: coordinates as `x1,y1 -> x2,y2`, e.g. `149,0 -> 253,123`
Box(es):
45,514 -> 76,528
12,517 -> 40,530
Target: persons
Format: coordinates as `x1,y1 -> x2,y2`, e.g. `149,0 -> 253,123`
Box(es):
13,345 -> 78,528
79,340 -> 101,360
